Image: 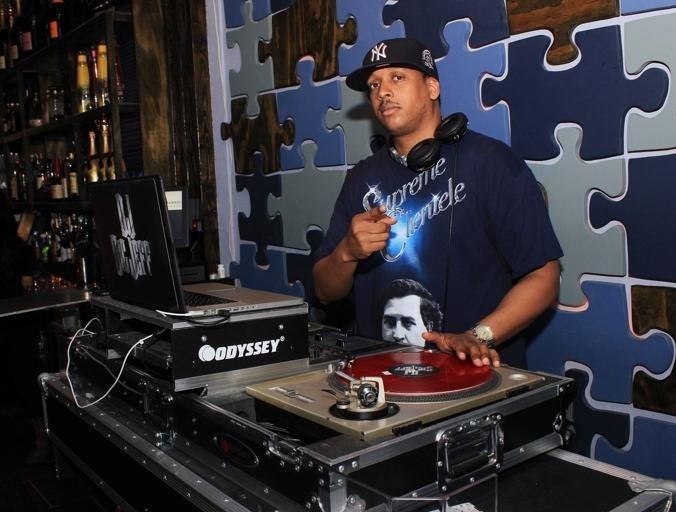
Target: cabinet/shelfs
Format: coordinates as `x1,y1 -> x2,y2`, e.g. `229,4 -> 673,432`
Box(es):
0,9 -> 143,209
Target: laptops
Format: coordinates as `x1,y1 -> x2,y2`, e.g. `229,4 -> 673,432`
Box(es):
84,175 -> 305,318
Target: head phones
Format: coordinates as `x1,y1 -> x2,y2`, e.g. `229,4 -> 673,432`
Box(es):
385,112 -> 468,171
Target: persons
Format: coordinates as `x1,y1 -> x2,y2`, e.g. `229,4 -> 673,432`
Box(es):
375,278 -> 444,349
0,188 -> 33,297
297,38 -> 565,372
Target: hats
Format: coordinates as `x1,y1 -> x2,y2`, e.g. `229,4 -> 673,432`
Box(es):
346,38 -> 439,91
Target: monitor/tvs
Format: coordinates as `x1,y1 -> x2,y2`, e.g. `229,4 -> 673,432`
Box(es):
163,186 -> 190,248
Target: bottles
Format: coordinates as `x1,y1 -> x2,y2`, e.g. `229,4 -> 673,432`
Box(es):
100,125 -> 116,180
0,150 -> 78,200
113,62 -> 125,101
34,214 -> 79,266
91,40 -> 110,108
76,51 -> 90,113
0,0 -> 67,71
87,131 -> 100,182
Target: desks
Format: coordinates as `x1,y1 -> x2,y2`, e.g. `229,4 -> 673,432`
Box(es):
39,370 -> 676,512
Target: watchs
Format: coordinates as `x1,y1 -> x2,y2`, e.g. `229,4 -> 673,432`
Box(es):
468,320 -> 495,348
